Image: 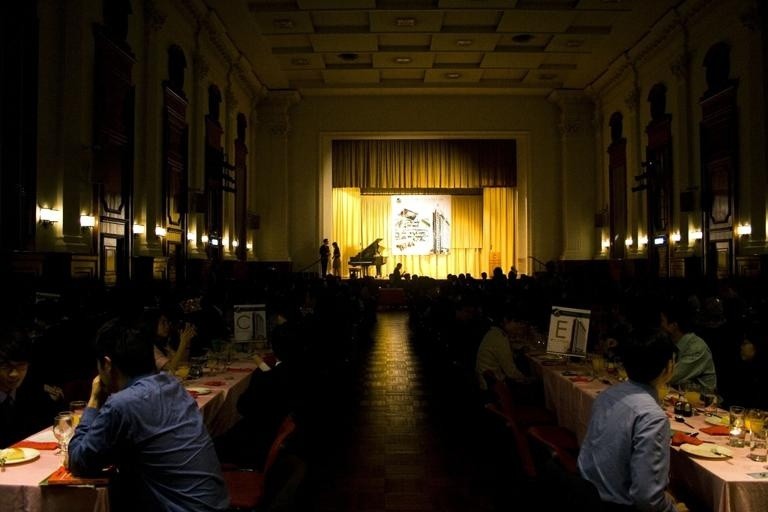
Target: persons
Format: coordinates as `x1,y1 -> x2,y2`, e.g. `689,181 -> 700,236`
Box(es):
318,238 -> 331,278
407,264 -> 766,511
0,263 -> 380,511
332,242 -> 341,277
384,263 -> 412,310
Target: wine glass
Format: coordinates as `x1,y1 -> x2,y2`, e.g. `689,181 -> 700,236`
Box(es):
54,401 -> 86,472
685,383 -> 701,416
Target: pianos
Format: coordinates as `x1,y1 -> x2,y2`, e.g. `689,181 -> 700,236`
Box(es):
348,239 -> 388,278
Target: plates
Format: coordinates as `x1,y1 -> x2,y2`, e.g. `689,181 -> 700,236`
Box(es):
679,442 -> 733,459
704,416 -> 729,427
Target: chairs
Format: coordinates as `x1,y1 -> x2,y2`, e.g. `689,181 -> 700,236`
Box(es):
481,379 -> 580,511
220,416 -> 297,511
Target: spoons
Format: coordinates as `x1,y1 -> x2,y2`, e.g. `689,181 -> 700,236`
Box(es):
675,415 -> 695,429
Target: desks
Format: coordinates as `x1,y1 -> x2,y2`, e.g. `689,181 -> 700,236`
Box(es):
508,333 -> 767,512
0,353 -> 274,511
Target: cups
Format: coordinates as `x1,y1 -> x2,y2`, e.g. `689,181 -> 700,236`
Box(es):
749,410 -> 768,462
704,388 -> 717,417
728,406 -> 746,448
